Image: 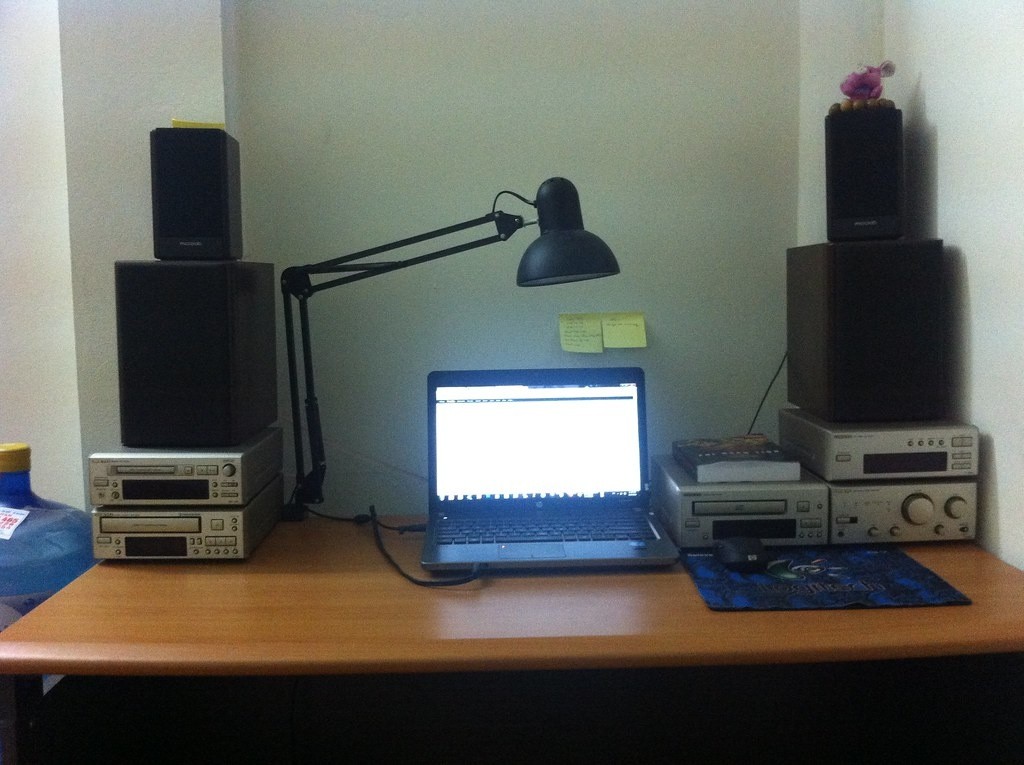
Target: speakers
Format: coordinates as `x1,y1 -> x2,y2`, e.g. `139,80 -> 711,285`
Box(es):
150,127 -> 243,262
785,238 -> 946,425
115,258 -> 278,448
825,107 -> 906,241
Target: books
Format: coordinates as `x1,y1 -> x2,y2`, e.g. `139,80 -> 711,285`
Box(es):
672,433 -> 801,482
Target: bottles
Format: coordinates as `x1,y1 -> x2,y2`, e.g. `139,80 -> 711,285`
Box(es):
0,442 -> 101,686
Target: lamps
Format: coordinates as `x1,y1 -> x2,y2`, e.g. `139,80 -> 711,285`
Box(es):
278,175 -> 620,522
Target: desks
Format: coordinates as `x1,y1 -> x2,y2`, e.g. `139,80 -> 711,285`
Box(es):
0,514 -> 1024,765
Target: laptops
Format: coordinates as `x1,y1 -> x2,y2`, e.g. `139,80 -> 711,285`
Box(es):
421,366 -> 681,571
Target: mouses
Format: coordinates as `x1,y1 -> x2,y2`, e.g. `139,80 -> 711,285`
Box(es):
713,537 -> 770,574
